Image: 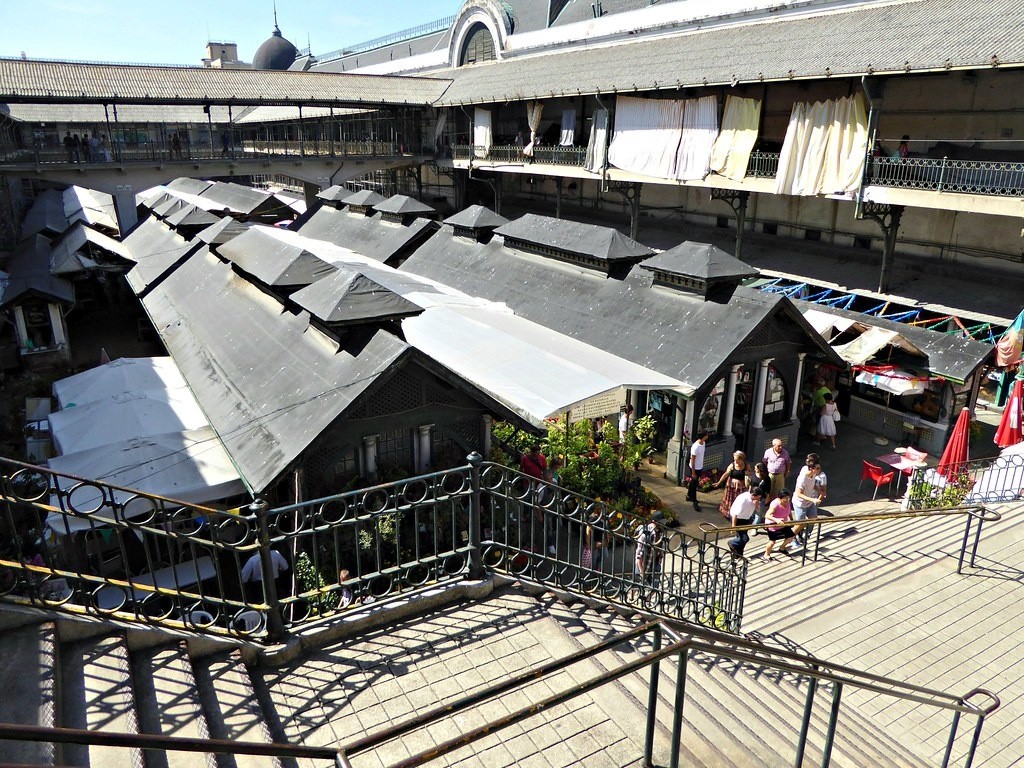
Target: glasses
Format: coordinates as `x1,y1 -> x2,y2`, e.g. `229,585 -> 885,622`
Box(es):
813,467 -> 821,470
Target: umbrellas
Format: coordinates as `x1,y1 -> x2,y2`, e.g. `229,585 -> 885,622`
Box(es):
855,369 -> 926,437
939,407 -> 970,494
993,380 -> 1024,447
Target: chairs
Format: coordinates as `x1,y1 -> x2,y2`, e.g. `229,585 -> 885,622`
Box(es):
891,446 -> 928,485
858,460 -> 895,500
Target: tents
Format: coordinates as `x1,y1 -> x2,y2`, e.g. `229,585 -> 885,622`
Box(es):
46,355 -> 247,537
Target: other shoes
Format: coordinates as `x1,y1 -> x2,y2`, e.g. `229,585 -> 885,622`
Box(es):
799,537 -> 805,543
727,542 -> 737,553
685,496 -> 692,501
779,548 -> 789,556
763,556 -> 773,562
693,504 -> 701,512
832,445 -> 835,448
813,441 -> 820,445
790,539 -> 800,545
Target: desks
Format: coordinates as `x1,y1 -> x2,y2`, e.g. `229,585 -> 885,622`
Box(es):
874,454 -> 917,493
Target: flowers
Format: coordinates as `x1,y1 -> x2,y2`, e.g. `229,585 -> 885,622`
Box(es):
685,466 -> 726,491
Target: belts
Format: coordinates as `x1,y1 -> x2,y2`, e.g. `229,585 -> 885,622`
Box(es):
773,473 -> 780,475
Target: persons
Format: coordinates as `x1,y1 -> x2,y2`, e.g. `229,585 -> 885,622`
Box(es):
586,507 -> 610,569
519,444 -> 547,522
763,488 -> 795,559
800,453 -> 827,505
727,487 -> 763,558
242,540 -> 288,605
337,571 -> 373,607
534,470 -> 563,554
685,429 -> 709,512
749,464 -> 772,536
809,378 -> 832,440
548,457 -> 562,480
634,511 -> 666,587
812,393 -> 838,450
712,451 -> 752,516
762,437 -> 791,501
791,458 -> 826,548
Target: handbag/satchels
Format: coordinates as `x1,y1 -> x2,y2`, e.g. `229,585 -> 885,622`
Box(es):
833,404 -> 841,421
